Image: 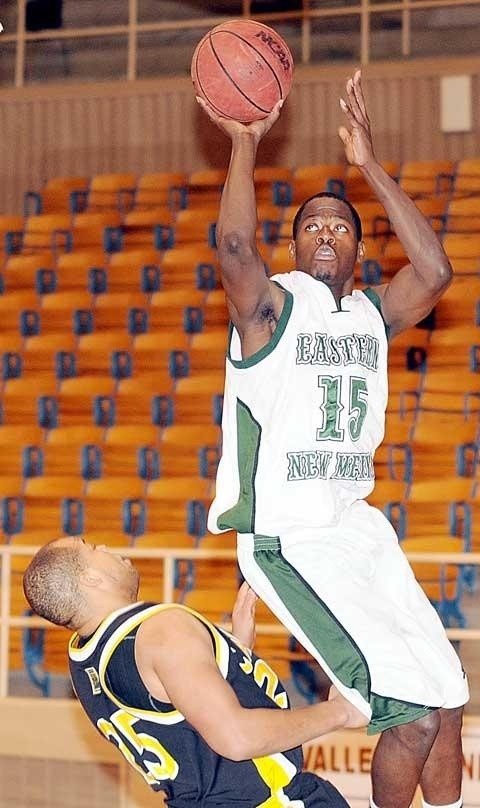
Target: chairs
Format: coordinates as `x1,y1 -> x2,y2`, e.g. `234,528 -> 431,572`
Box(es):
3,155 -> 479,704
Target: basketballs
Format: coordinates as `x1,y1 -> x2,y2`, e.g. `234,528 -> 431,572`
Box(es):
191,20 -> 295,123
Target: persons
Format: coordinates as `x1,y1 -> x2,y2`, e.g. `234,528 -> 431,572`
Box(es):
23,536 -> 372,808
195,68 -> 470,808
